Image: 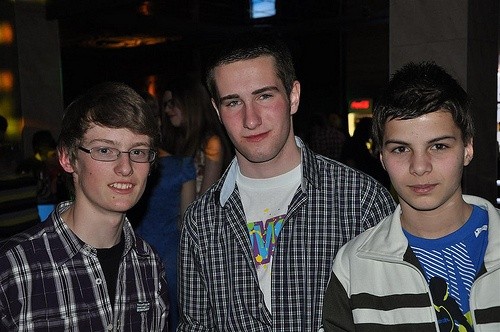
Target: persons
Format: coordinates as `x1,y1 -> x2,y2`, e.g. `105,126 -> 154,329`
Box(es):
0,83 -> 171,332
116,75 -> 226,332
176,36 -> 399,332
1,109 -> 87,247
322,63 -> 496,332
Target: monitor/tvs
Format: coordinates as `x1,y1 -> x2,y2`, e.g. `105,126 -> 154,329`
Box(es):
248,0 -> 278,20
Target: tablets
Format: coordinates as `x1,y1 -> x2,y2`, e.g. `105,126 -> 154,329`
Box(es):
36,203 -> 56,222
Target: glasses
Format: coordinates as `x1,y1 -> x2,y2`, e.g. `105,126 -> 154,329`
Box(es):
162,97 -> 175,110
78,145 -> 157,163
